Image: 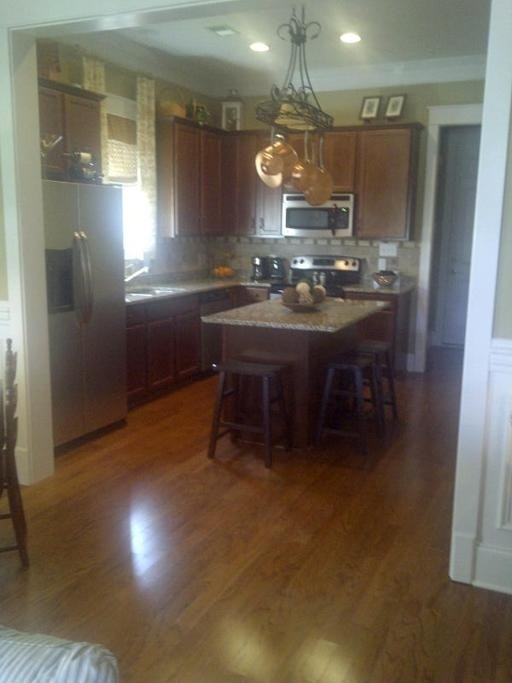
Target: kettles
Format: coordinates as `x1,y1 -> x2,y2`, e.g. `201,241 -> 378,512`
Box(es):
268,254 -> 285,279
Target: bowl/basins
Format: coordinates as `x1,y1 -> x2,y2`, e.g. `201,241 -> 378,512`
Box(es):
372,270 -> 398,286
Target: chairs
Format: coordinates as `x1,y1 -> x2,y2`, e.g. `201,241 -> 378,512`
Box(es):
1,339 -> 32,580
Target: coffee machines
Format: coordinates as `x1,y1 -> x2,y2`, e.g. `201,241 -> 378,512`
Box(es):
249,255 -> 266,281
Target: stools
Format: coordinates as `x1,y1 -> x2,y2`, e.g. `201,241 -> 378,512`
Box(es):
307,338 -> 397,461
206,355 -> 294,469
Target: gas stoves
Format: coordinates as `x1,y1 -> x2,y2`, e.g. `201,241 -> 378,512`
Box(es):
269,278 -> 359,295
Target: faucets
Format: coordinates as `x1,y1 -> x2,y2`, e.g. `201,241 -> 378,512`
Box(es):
124,265 -> 150,284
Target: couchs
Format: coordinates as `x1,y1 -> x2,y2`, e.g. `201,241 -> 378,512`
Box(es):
0,623 -> 122,683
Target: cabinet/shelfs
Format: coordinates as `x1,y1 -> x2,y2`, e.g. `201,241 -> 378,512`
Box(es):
225,133 -> 283,238
37,82 -> 108,185
201,289 -> 237,371
125,301 -> 149,392
236,286 -> 269,307
351,125 -> 420,241
149,292 -> 201,386
282,128 -> 355,194
154,116 -> 225,242
343,289 -> 411,378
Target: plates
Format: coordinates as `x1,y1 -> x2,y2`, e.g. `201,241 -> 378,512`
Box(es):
282,301 -> 320,311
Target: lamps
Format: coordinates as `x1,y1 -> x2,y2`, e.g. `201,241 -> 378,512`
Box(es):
254,4 -> 338,212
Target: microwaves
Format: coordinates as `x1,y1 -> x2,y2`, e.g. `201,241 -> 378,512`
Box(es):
281,191 -> 356,238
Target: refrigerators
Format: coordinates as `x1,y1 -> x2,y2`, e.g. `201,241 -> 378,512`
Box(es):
38,176 -> 129,446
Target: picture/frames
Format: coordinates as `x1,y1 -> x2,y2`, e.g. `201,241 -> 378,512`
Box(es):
384,94 -> 408,120
358,95 -> 382,120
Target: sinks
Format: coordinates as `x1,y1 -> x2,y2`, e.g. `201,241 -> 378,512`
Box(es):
127,287 -> 186,295
126,293 -> 150,305
